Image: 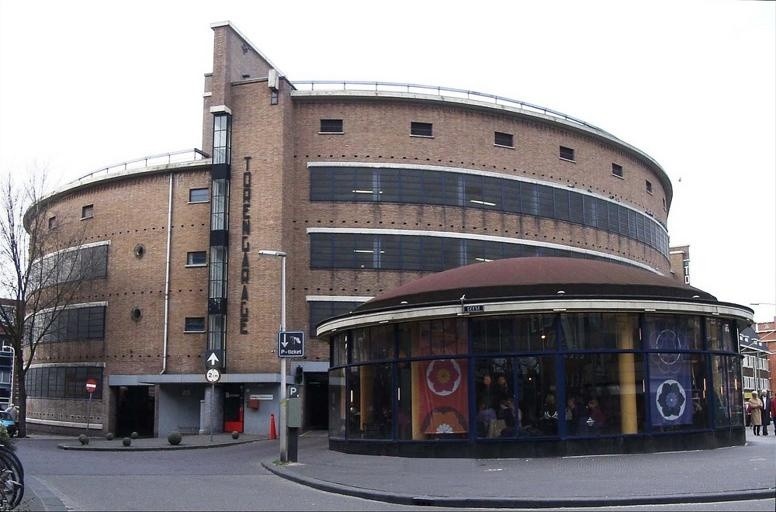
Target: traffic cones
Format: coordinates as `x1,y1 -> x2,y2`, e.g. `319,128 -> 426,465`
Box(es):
266,413 -> 276,440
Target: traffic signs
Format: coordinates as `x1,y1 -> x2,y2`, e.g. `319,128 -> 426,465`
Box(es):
277,330 -> 304,358
205,366 -> 221,384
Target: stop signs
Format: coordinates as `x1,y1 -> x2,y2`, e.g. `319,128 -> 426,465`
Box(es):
84,377 -> 96,393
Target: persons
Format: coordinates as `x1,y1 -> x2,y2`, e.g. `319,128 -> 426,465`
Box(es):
477,374 -> 609,436
4,403 -> 15,414
748,390 -> 764,436
770,393 -> 776,436
760,389 -> 772,435
743,398 -> 752,427
8,405 -> 20,437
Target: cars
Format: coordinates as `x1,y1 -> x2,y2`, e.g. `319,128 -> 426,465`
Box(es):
1,401 -> 20,440
745,401 -> 752,427
717,403 -> 742,426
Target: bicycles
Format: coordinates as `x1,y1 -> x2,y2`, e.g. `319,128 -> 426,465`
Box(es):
0,442 -> 25,512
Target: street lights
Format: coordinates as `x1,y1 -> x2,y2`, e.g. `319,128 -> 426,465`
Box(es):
749,301 -> 775,306
258,246 -> 290,465
2,344 -> 15,409
